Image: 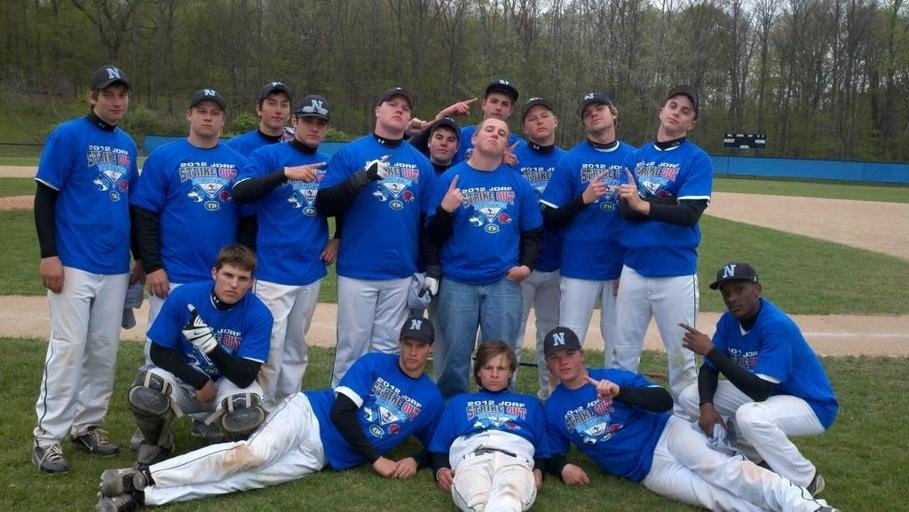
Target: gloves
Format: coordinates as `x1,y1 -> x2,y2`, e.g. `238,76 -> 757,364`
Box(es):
181,304 -> 217,356
364,154 -> 391,180
421,276 -> 440,302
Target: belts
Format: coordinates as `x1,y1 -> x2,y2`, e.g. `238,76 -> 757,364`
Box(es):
463,447 -> 534,462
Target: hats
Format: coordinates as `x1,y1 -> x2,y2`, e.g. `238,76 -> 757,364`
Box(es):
708,264 -> 760,290
398,317 -> 436,344
543,326 -> 583,356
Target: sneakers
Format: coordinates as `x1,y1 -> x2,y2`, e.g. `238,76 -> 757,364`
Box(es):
191,419 -> 225,439
92,488 -> 145,512
807,471 -> 826,498
128,428 -> 177,466
71,425 -> 121,459
813,495 -> 841,512
99,466 -> 152,498
31,443 -> 72,475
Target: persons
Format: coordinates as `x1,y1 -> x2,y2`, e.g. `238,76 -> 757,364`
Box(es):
676,263 -> 840,496
312,77 -> 713,427
126,84 -> 259,454
542,326 -> 838,512
128,243 -> 275,469
97,315 -> 444,511
227,94 -> 346,418
226,83 -> 294,247
426,342 -> 549,512
30,60 -> 139,473
